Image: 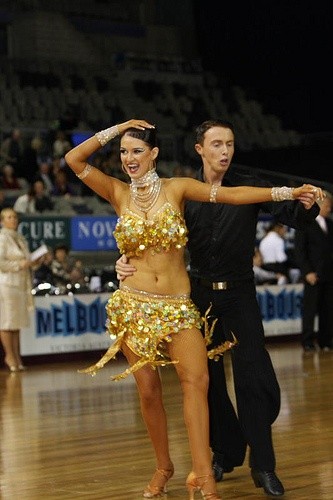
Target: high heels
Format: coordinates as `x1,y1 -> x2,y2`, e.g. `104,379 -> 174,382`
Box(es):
144,466 -> 174,497
3,358 -> 18,372
18,363 -> 25,370
186,471 -> 221,500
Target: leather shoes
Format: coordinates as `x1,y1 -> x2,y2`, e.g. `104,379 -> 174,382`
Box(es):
212,458 -> 234,481
251,469 -> 284,496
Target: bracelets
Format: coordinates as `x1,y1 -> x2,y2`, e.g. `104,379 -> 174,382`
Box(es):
95,125 -> 120,146
271,186 -> 295,201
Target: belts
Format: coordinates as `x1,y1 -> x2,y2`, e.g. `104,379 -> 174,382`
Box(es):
197,279 -> 240,290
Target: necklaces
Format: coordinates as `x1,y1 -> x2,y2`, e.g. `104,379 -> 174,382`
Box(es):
130,168 -> 161,213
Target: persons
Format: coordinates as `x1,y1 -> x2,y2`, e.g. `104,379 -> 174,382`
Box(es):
115,119 -> 321,498
0,126 -> 132,297
252,180 -> 333,353
155,163 -> 195,178
65,118 -> 327,500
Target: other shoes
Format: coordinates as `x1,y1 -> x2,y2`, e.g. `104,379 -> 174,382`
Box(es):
303,344 -> 315,352
